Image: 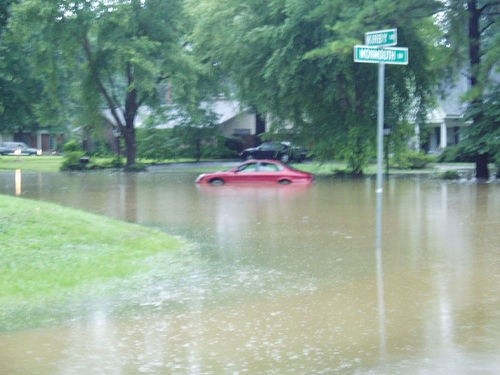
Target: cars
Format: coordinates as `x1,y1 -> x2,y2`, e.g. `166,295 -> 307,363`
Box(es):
0,142 -> 42,155
239,140 -> 308,164
195,159 -> 315,184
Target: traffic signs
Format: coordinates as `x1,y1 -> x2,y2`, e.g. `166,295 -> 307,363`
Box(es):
364,28 -> 397,48
353,46 -> 408,66
